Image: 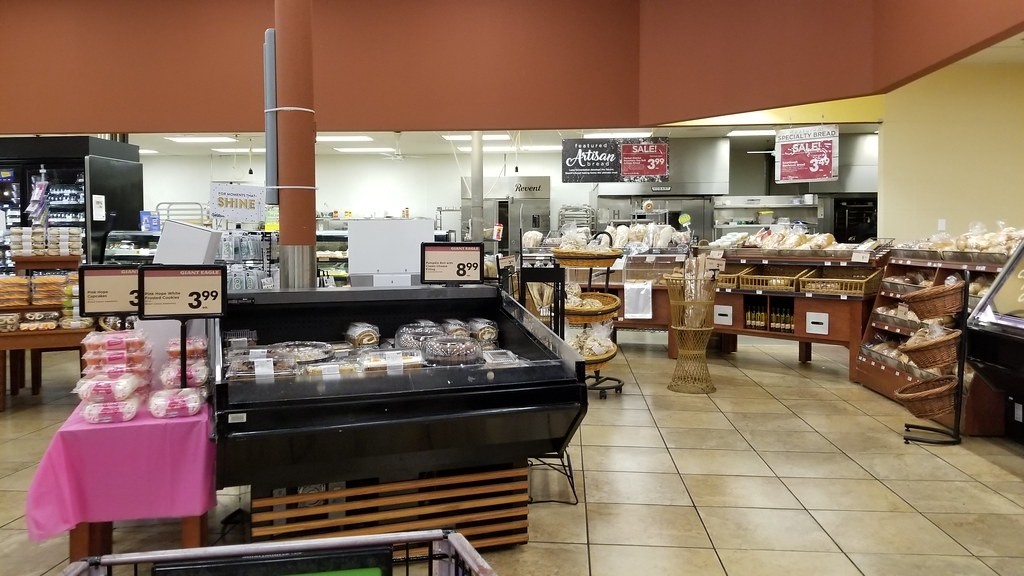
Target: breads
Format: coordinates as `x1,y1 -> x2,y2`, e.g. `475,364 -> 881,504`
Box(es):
870,231 -> 1024,392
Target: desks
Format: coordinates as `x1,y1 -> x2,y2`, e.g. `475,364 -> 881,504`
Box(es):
27,387 -> 219,565
0,254 -> 96,414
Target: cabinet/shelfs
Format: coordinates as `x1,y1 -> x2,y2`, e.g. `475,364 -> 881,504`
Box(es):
690,246 -> 892,384
101,216 -> 435,287
711,194 -> 820,243
560,252 -> 688,359
851,255 -> 1010,437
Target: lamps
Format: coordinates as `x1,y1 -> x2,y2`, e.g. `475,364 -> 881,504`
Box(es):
248,138 -> 254,175
514,148 -> 518,173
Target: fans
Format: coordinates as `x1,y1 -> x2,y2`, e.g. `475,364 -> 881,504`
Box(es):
376,131 -> 424,160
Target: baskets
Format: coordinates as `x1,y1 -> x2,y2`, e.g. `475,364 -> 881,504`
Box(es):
564,292 -> 621,324
894,375 -> 959,419
799,268 -> 883,294
739,265 -> 814,292
585,342 -> 618,373
551,246 -> 622,267
904,281 -> 965,321
717,266 -> 754,287
897,329 -> 962,370
